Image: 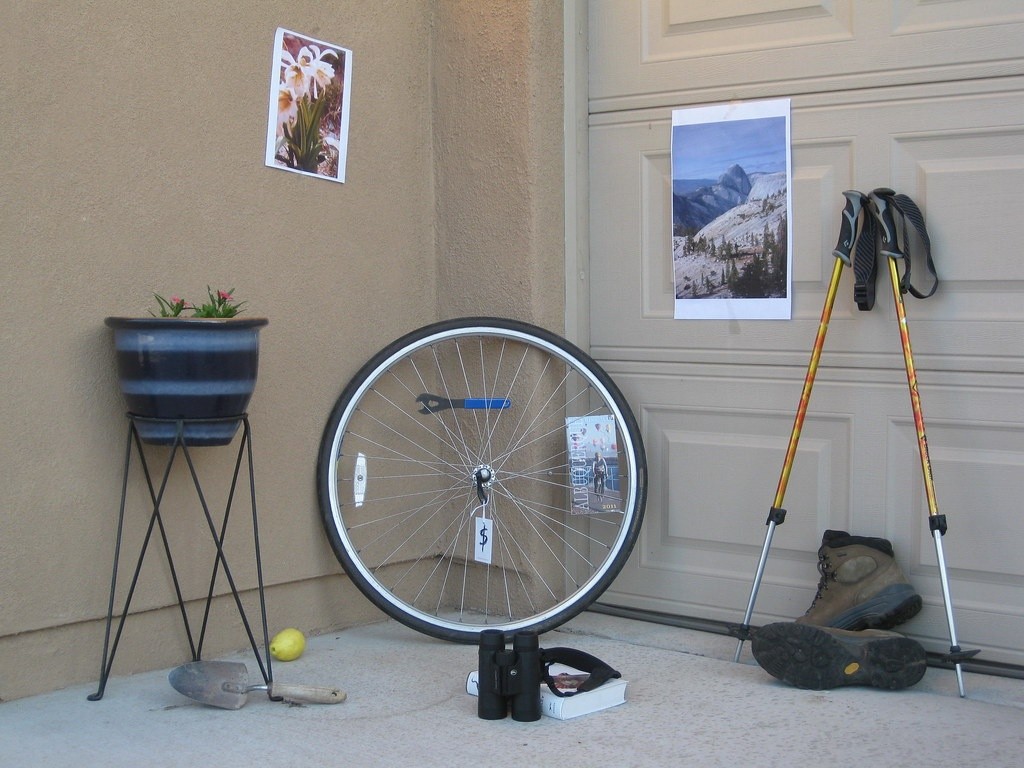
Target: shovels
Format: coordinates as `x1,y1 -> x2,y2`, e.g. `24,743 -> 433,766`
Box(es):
168,661 -> 348,711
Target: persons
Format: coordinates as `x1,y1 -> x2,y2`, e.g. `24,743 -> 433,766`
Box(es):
592,452 -> 608,497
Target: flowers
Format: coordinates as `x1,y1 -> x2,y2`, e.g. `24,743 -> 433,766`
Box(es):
276,44 -> 339,175
146,284 -> 247,318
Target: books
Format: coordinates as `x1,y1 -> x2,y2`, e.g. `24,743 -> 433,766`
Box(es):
466,661 -> 628,720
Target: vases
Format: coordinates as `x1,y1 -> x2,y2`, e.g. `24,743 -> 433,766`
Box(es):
105,317 -> 268,446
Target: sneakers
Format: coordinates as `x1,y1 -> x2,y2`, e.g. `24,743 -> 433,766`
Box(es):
794,530 -> 923,630
751,621 -> 927,692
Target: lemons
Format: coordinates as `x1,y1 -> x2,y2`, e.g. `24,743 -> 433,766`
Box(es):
270,628 -> 305,661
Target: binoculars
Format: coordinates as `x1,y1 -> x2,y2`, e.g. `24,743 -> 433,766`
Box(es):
477,629 -> 542,722
